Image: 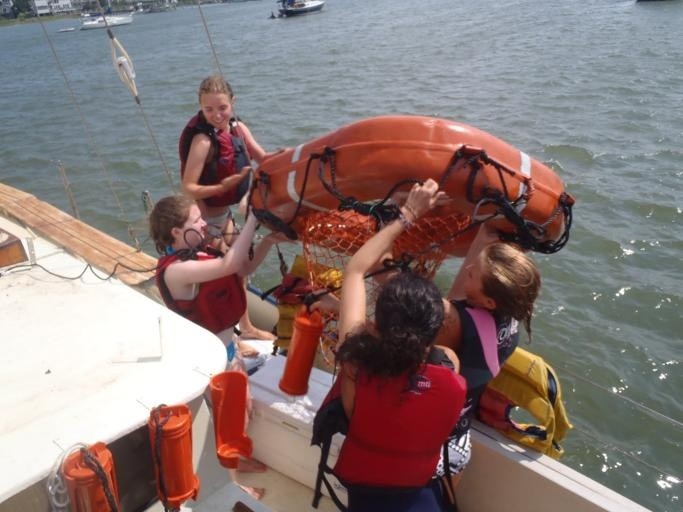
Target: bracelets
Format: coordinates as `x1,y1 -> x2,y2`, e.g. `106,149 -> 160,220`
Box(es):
403,201 -> 420,221
395,211 -> 412,232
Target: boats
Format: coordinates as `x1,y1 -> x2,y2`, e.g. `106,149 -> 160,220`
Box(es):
261,253 -> 577,466
0,181 -> 665,512
237,110 -> 578,256
268,0 -> 326,20
55,0 -> 151,33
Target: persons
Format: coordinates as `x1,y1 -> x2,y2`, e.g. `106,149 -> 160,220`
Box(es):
177,73 -> 293,358
148,191 -> 298,502
368,211 -> 541,505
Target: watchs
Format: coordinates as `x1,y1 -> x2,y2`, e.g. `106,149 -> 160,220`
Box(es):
330,177 -> 468,511
